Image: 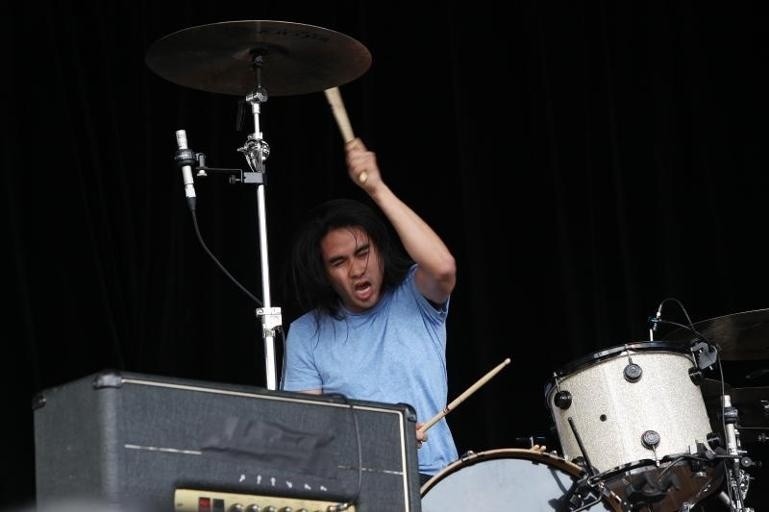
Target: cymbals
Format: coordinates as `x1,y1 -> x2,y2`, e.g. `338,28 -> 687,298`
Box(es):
141,20 -> 371,98
665,310 -> 768,358
715,384 -> 769,429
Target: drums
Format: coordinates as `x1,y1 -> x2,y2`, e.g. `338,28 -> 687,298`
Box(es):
544,342 -> 724,511
418,448 -> 629,512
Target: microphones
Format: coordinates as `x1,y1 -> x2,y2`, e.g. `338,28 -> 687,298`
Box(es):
174,128 -> 197,211
648,304 -> 664,340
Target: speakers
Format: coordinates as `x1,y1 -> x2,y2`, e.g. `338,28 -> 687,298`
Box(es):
32,368 -> 422,511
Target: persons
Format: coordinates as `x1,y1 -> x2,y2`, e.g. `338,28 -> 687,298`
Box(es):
279,137 -> 461,486
